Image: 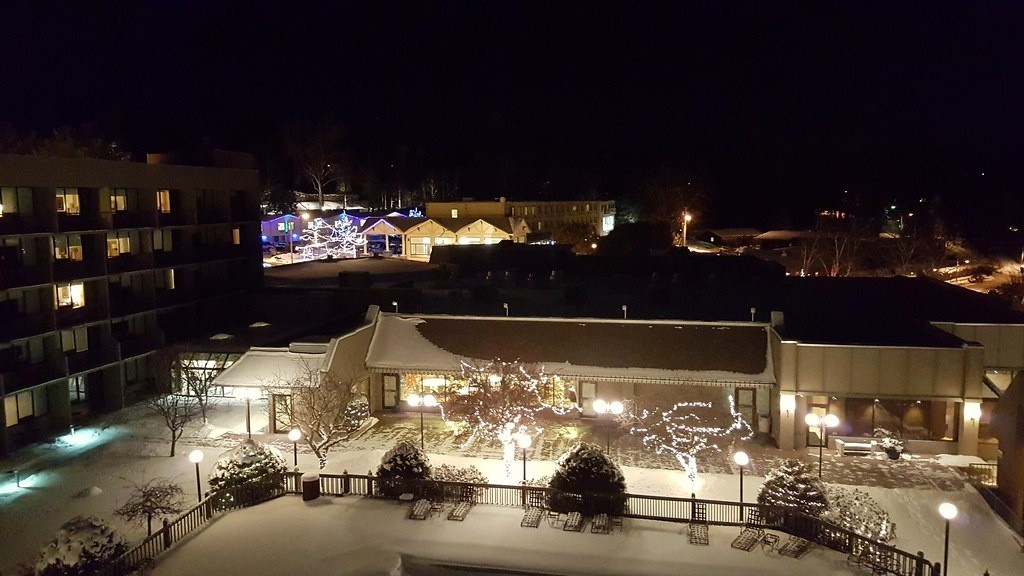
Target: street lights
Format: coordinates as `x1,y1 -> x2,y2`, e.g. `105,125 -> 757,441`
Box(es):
733,451 -> 748,521
288,429 -> 301,492
188,449 -> 204,516
516,434 -> 531,481
407,393 -> 436,450
592,398 -> 625,456
956,259 -> 970,283
939,502 -> 959,576
805,413 -> 840,481
684,215 -> 692,247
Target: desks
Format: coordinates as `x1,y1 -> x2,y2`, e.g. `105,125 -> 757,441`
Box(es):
868,555 -> 902,576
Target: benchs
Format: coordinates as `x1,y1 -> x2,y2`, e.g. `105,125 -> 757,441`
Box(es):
835,439 -> 877,456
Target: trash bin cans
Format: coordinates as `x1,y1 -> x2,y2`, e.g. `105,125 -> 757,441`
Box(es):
301,473 -> 322,501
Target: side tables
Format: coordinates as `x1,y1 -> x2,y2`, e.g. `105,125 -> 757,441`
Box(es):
761,533 -> 780,552
547,509 -> 561,521
399,493 -> 416,506
429,504 -> 444,518
611,518 -> 623,532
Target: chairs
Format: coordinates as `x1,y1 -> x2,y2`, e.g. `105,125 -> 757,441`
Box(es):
591,513 -> 610,534
730,509 -> 765,552
778,530 -> 817,559
847,543 -> 870,569
448,484 -> 474,520
688,504 -> 708,544
522,489 -> 545,529
410,486 -> 432,520
563,492 -> 584,532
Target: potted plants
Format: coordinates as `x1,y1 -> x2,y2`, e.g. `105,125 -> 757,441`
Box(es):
874,427 -> 913,459
